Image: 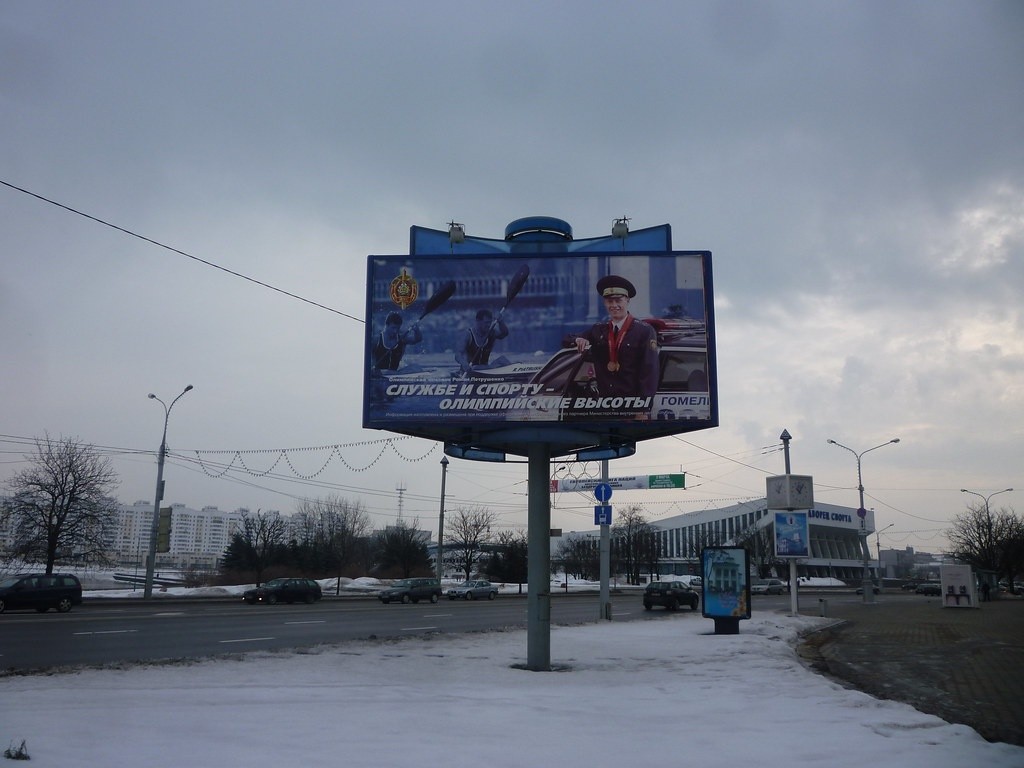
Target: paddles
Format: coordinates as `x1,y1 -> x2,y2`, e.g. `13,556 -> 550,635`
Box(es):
460,263 -> 531,380
370,279 -> 457,373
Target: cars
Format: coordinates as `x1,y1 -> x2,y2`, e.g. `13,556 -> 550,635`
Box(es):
902,577 -> 1024,597
749,578 -> 786,595
447,579 -> 499,601
856,584 -> 880,595
642,580 -> 699,611
379,576 -> 442,604
689,577 -> 703,585
0,572 -> 83,614
506,317 -> 712,420
243,576 -> 324,606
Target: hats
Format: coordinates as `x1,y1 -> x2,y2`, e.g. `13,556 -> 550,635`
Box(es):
597,275 -> 636,298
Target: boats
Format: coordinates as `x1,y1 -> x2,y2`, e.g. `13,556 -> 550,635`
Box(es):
382,360 -> 544,384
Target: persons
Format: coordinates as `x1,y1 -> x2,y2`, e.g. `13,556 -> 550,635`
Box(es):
560,274 -> 660,420
454,308 -> 508,374
372,311 -> 423,374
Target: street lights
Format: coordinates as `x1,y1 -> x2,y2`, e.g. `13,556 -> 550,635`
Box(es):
875,523 -> 894,582
826,438 -> 900,601
960,488 -> 1015,566
142,384 -> 193,601
